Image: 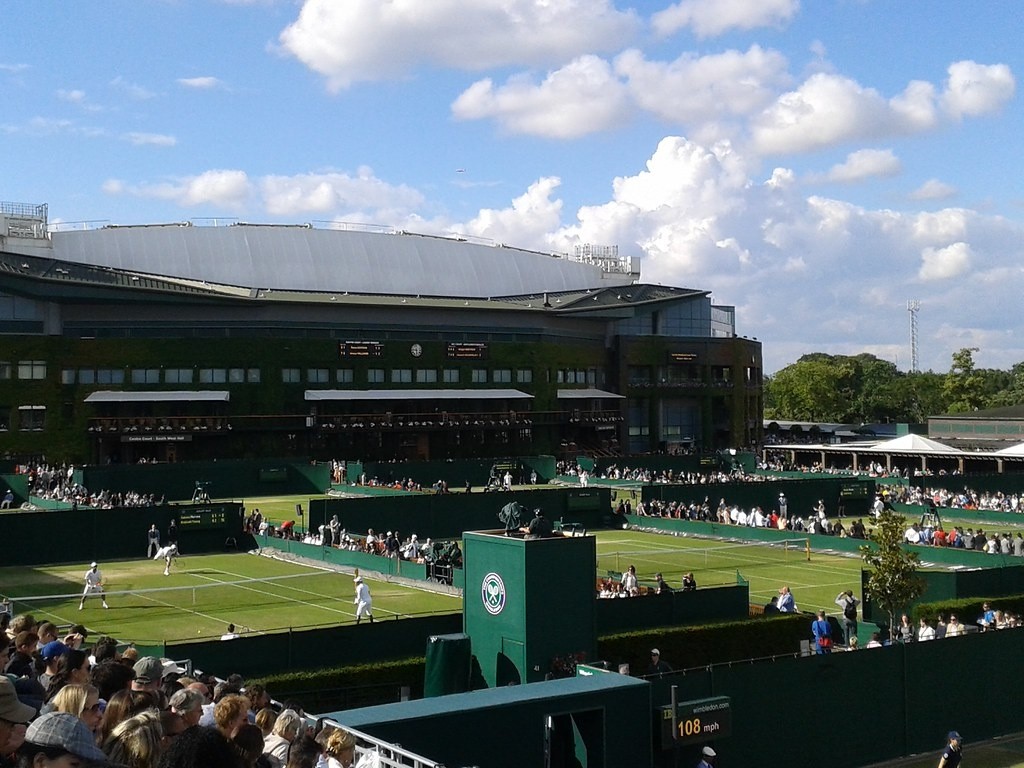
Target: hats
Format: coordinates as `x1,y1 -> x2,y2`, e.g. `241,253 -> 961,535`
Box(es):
41,641 -> 70,661
133,657 -> 164,684
24,711 -> 109,760
91,562 -> 98,567
162,661 -> 186,678
703,746 -> 716,756
0,676 -> 37,722
654,573 -> 662,576
652,648 -> 660,654
353,576 -> 363,582
949,731 -> 963,740
533,508 -> 543,514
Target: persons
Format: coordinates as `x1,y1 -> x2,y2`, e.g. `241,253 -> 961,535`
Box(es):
242,508 -> 463,568
731,432 -> 1024,513
616,495 -> 732,524
1,489 -> 13,509
697,746 -> 716,768
606,463 -> 729,484
598,565 -> 696,599
558,462 -> 601,487
0,598 -> 356,768
518,507 -> 553,538
78,562 -> 108,610
137,456 -> 159,464
354,576 -> 374,624
642,649 -> 673,675
904,522 -> 1024,557
499,501 -> 526,538
193,482 -> 211,504
763,585 -> 1024,654
148,518 -> 180,576
482,468 -> 513,492
312,460 -> 449,494
520,464 -> 536,484
466,479 -> 471,493
730,505 -> 873,539
939,732 -> 964,768
15,456 -> 155,509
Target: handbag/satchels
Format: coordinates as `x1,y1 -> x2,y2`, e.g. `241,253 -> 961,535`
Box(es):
819,636 -> 833,648
258,743 -> 289,768
896,626 -> 902,640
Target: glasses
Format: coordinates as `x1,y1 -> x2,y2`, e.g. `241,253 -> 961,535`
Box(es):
84,703 -> 100,713
84,665 -> 91,673
651,654 -> 657,656
204,681 -> 217,686
203,692 -> 209,697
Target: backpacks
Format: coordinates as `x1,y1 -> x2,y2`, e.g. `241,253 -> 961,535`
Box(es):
844,599 -> 857,621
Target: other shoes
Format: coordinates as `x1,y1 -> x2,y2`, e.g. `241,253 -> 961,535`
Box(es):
103,603 -> 108,608
79,606 -> 84,610
164,571 -> 170,576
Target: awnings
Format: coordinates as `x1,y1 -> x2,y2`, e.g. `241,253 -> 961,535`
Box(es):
83,390 -> 626,401
